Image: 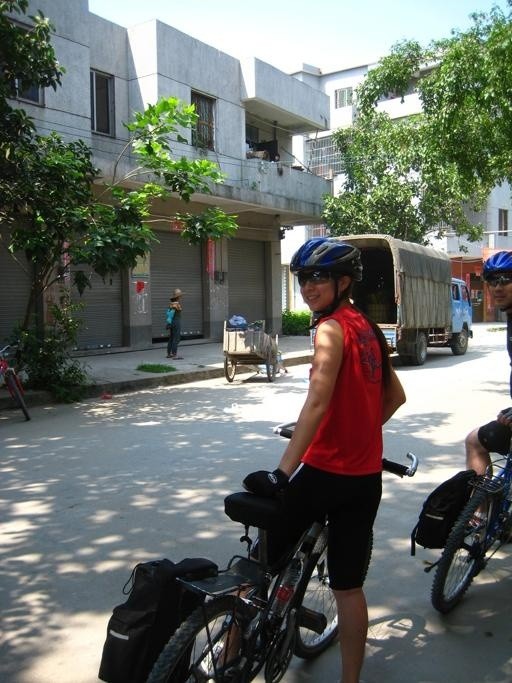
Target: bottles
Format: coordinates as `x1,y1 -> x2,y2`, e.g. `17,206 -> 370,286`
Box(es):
271,550 -> 305,618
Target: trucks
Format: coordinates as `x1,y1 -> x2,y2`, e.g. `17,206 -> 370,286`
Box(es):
309,233 -> 476,367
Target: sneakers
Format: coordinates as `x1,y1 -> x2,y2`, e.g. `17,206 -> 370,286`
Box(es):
167,353 -> 184,360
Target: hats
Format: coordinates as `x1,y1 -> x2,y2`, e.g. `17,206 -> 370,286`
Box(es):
168,288 -> 186,300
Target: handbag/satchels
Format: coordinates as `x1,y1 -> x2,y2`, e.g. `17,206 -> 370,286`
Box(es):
98,557 -> 219,683
415,470 -> 477,549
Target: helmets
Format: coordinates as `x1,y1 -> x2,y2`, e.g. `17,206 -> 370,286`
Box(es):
481,251 -> 512,281
290,237 -> 364,282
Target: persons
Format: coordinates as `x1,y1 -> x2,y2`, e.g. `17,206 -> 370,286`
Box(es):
164,288 -> 186,359
202,237 -> 406,682
465,251 -> 512,533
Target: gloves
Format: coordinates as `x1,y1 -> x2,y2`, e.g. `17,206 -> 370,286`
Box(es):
242,468 -> 290,497
500,406 -> 511,421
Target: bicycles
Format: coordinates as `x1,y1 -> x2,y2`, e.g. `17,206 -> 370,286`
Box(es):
0,339 -> 33,422
424,427 -> 511,615
141,419 -> 419,683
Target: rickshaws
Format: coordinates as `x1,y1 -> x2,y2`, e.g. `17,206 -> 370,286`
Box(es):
221,318 -> 289,383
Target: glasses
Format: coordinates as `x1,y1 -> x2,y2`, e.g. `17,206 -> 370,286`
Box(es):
486,274 -> 511,287
298,270 -> 349,287
